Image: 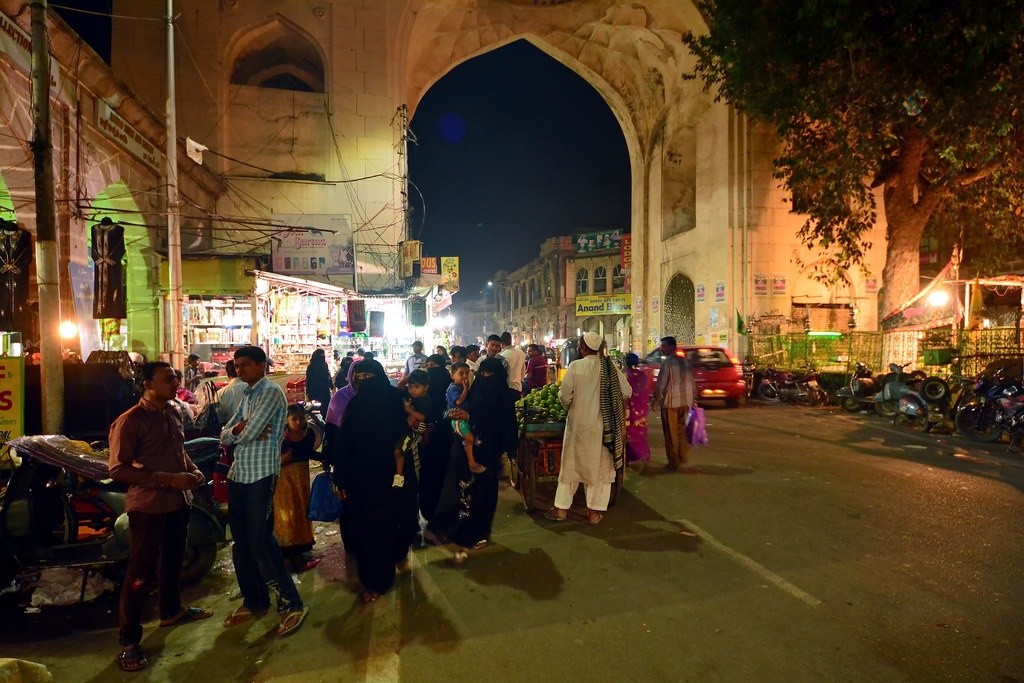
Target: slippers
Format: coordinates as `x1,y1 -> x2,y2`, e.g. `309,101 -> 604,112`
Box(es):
276,606 -> 310,636
469,463 -> 486,473
158,606 -> 213,628
116,645 -> 150,671
545,504 -> 566,521
223,606 -> 269,628
588,509 -> 604,525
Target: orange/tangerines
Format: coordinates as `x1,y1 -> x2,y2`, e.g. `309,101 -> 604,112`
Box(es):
514,381 -> 567,429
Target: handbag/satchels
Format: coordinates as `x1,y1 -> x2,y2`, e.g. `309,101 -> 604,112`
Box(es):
685,407 -> 708,449
307,469 -> 342,523
193,380 -> 231,436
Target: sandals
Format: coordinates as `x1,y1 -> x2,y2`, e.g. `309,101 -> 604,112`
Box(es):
451,544 -> 468,565
473,538 -> 486,549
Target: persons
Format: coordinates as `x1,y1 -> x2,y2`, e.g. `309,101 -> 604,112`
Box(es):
339,358 -> 415,605
651,335 -> 694,472
544,331 -> 634,526
217,344 -> 310,639
107,360 -> 214,674
107,327 -> 695,674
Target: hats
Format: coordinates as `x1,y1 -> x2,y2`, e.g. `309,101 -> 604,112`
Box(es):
584,332 -> 602,350
626,353 -> 639,367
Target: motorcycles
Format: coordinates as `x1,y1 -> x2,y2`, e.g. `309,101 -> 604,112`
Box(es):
0,434 -> 234,634
741,351 -> 1024,454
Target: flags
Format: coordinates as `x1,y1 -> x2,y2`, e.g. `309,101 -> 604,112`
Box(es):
736,312 -> 748,335
879,259 -> 963,334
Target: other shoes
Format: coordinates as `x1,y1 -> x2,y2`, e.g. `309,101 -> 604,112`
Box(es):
301,559 -> 321,571
362,591 -> 380,602
667,459 -> 680,471
397,558 -> 410,572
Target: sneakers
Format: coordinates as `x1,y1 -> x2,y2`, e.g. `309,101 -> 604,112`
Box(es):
392,474 -> 405,488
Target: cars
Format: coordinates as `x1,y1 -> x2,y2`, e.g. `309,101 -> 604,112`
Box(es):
637,341 -> 746,411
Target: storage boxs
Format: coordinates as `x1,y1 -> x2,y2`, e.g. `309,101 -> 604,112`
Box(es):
923,349 -> 952,366
